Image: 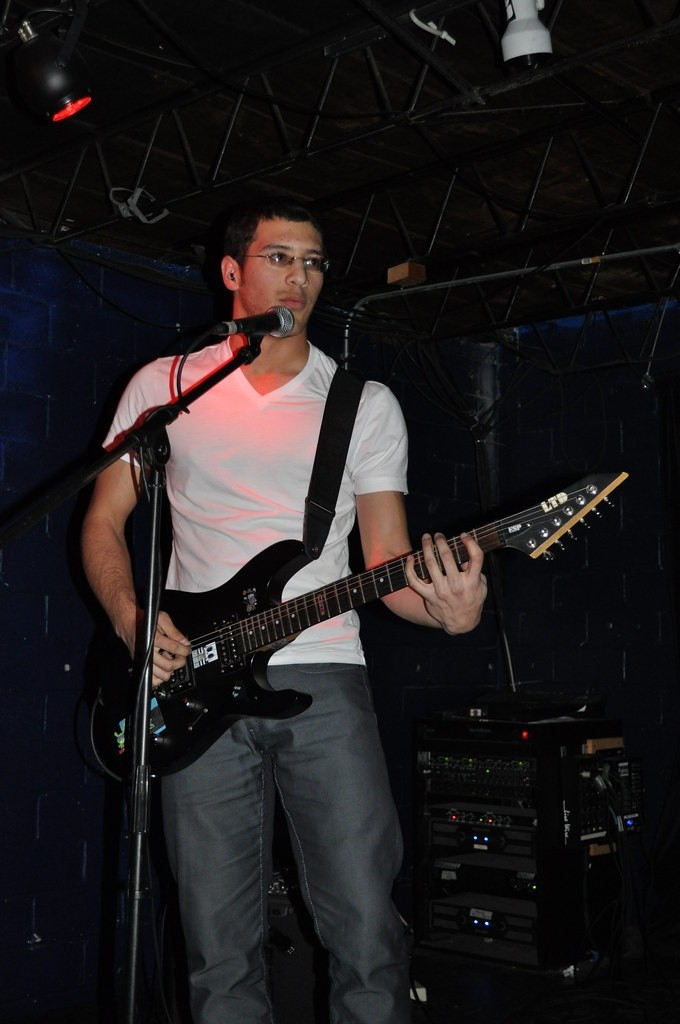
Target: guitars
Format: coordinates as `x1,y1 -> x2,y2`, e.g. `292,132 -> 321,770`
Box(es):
87,464 -> 633,785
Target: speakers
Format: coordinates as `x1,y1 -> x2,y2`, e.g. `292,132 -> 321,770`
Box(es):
262,896 -> 333,1024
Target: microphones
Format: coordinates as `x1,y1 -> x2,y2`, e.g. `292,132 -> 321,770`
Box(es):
214,305 -> 296,338
264,925 -> 297,956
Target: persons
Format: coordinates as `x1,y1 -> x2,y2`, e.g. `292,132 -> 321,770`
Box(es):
82,193 -> 487,1024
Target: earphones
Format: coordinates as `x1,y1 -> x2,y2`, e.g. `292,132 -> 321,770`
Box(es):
229,270 -> 236,282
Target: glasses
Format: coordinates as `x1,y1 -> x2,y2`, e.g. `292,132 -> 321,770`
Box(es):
235,252 -> 329,274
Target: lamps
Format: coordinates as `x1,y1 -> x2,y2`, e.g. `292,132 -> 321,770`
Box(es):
15,8 -> 91,124
503,0 -> 553,69
